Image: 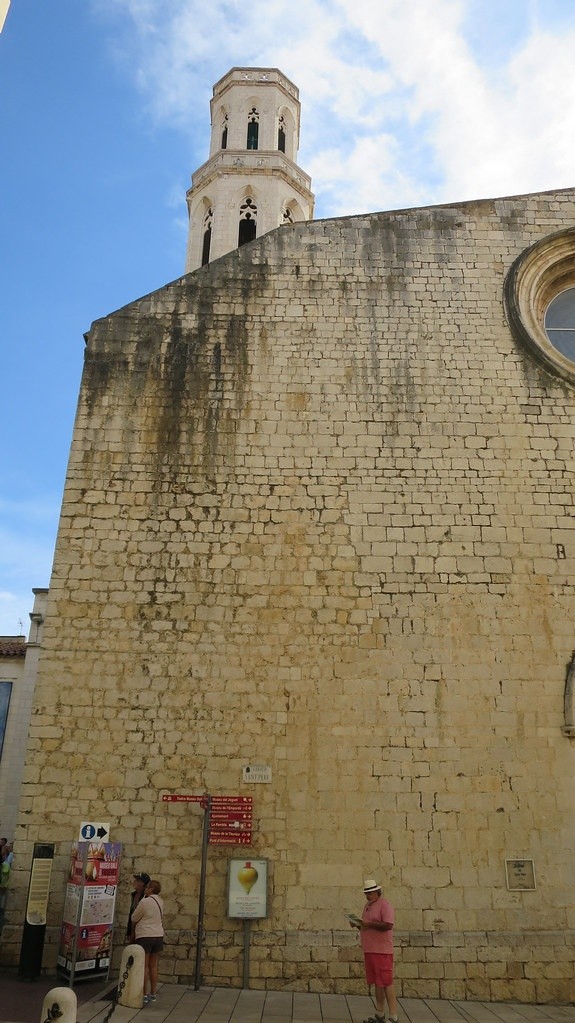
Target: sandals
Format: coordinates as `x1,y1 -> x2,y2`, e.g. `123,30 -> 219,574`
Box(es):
389,1018 -> 400,1023
363,1014 -> 386,1023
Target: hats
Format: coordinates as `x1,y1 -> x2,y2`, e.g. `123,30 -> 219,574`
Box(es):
133,873 -> 150,885
363,880 -> 382,893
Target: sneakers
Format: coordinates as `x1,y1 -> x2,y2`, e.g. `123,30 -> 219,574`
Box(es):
144,996 -> 149,1003
149,995 -> 157,1002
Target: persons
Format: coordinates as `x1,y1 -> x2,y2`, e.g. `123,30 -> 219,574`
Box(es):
0,838 -> 13,913
127,873 -> 164,1003
349,880 -> 398,1023
